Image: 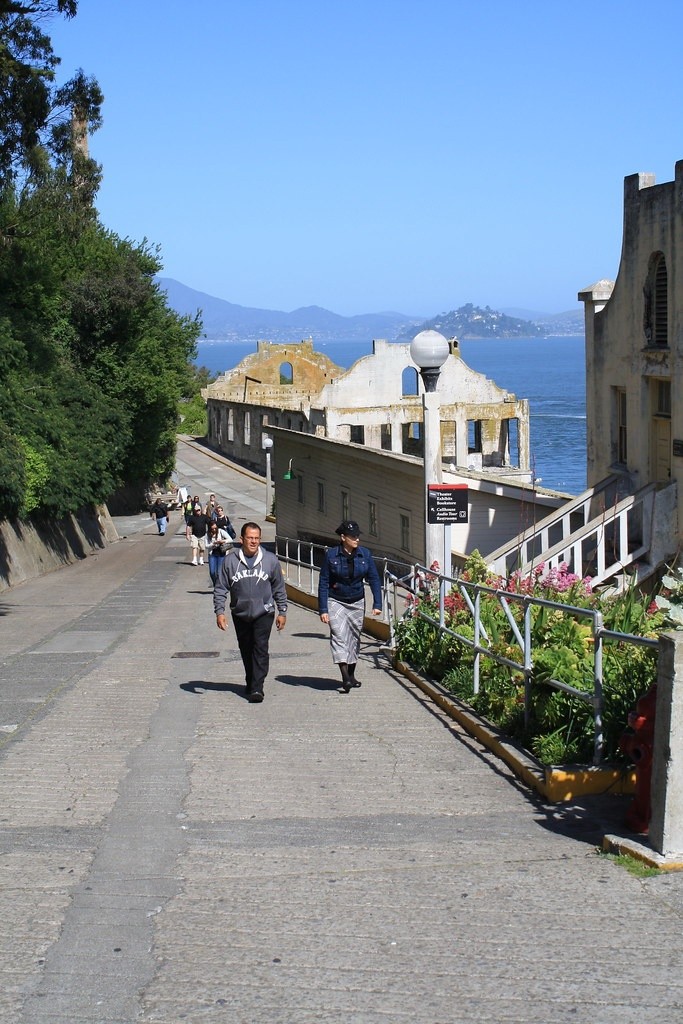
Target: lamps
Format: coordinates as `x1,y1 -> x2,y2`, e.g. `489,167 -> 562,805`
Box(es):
283,455 -> 309,480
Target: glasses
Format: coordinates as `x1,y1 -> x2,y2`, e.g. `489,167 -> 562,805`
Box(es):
195,498 -> 199,499
342,523 -> 359,530
195,509 -> 201,512
242,537 -> 262,542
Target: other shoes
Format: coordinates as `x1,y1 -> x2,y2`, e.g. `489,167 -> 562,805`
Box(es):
250,690 -> 263,703
160,532 -> 164,536
349,678 -> 360,687
343,682 -> 352,692
199,560 -> 204,565
245,676 -> 251,693
191,560 -> 198,565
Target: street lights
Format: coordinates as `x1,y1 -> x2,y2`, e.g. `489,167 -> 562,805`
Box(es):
263,436 -> 274,516
409,329 -> 449,596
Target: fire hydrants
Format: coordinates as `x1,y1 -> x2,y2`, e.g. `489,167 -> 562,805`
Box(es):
618,686 -> 656,832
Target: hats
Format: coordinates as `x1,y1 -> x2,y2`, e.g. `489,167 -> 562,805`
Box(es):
340,520 -> 361,536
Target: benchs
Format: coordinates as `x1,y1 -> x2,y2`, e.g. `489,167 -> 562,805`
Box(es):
147,493 -> 179,511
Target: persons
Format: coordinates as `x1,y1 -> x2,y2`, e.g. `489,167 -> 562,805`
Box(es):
318,521 -> 382,691
213,522 -> 288,702
182,494 -> 230,566
150,498 -> 168,536
206,520 -> 233,588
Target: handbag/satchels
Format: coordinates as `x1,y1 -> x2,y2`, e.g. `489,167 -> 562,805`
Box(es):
226,524 -> 236,540
218,543 -> 234,557
